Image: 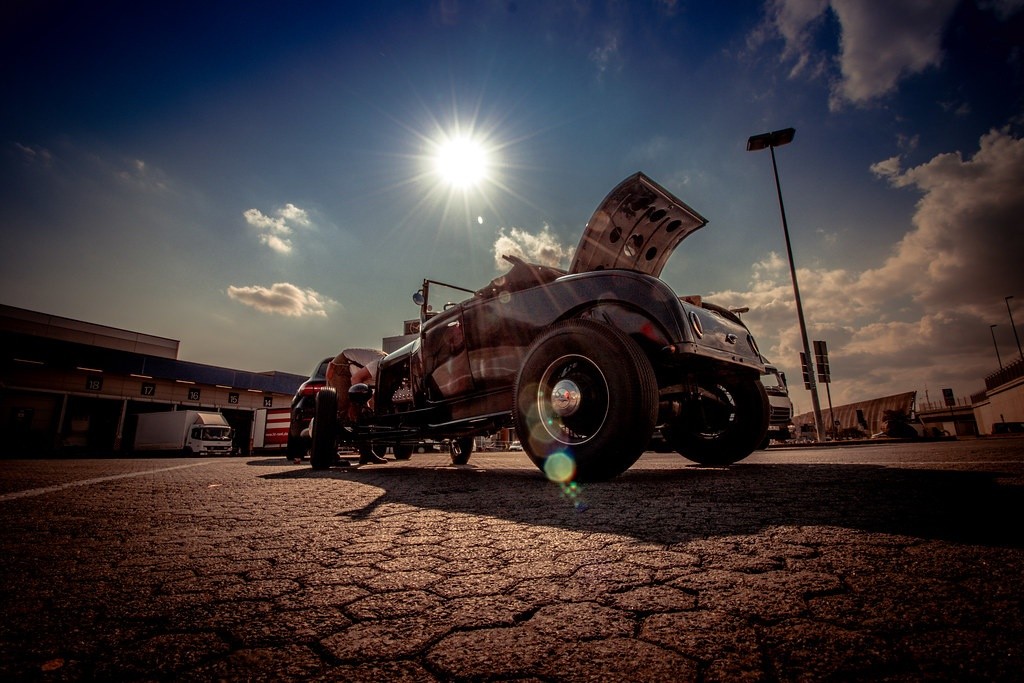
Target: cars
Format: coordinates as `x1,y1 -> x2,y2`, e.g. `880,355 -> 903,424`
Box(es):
508,441 -> 523,452
286,355 -> 415,462
380,172 -> 771,486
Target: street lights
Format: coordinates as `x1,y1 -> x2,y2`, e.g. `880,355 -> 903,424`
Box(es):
730,307 -> 749,322
1004,295 -> 1024,361
989,324 -> 1003,369
747,125 -> 827,441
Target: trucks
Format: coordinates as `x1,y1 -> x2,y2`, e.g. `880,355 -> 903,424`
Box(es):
756,363 -> 797,451
134,410 -> 236,457
